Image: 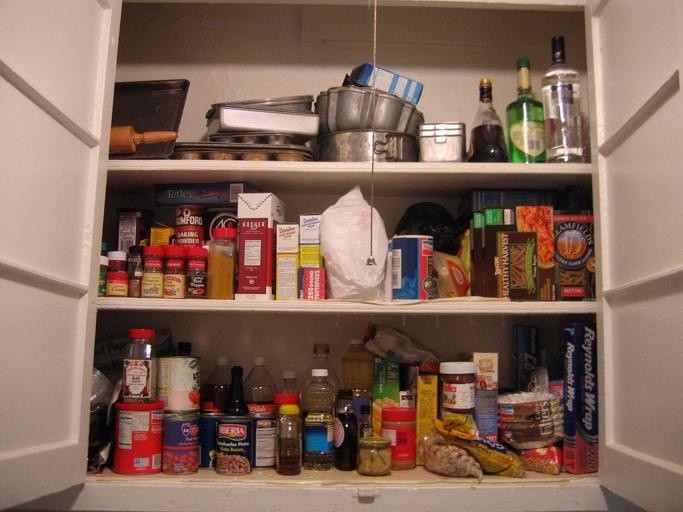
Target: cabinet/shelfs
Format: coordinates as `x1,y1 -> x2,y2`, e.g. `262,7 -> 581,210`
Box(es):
86,0 -> 600,512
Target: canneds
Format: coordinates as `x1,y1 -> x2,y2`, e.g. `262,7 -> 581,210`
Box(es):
439,362 -> 476,412
162,411 -> 255,475
176,204 -> 205,244
392,234 -> 434,300
100,244 -> 207,298
383,408 -> 417,472
358,438 -> 392,476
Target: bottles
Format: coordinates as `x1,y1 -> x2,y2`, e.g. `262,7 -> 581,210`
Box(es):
126,244 -> 143,297
119,327 -> 391,477
468,76 -> 507,162
539,35 -> 584,163
504,57 -> 546,162
98,256 -> 109,297
163,245 -> 186,298
105,252 -> 127,296
140,246 -> 162,297
185,246 -> 207,298
207,226 -> 237,299
